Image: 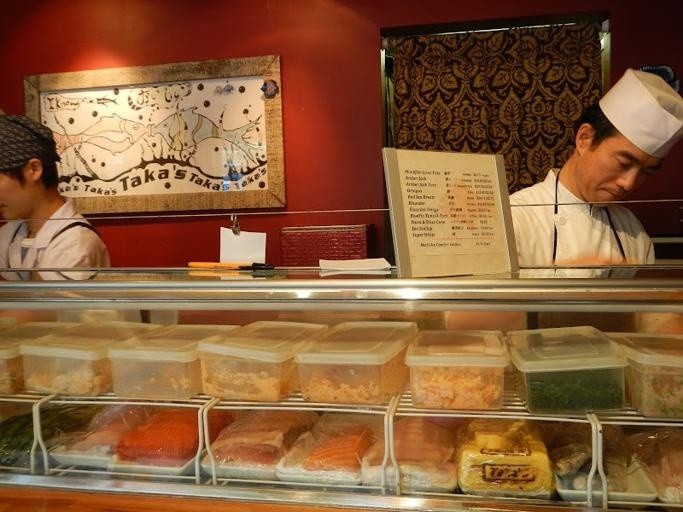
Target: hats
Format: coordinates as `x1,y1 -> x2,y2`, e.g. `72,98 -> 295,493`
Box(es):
0,113 -> 59,169
598,66 -> 682,157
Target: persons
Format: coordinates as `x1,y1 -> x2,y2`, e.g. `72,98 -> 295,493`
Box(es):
0,113 -> 111,282
444,69 -> 683,333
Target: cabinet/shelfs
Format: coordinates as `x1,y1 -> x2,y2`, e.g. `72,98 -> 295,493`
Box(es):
1,268 -> 681,512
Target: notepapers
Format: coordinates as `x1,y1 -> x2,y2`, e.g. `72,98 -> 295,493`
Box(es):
318,256 -> 392,272
318,272 -> 393,277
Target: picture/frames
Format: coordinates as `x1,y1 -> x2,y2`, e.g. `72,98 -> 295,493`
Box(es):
21,50 -> 288,217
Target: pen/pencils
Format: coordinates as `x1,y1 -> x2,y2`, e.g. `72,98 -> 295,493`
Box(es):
188,261 -> 274,270
189,271 -> 275,276
